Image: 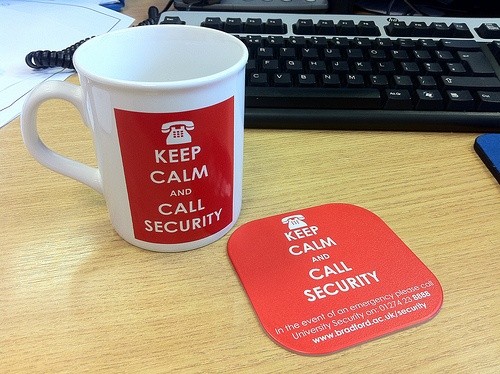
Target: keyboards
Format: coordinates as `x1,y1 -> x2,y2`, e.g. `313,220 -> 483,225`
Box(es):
157,11 -> 500,135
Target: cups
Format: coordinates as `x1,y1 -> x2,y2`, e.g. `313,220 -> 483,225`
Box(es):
21,25 -> 249,253
